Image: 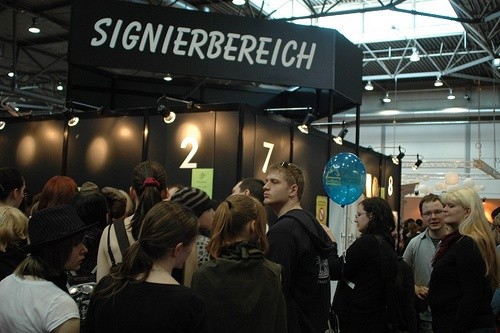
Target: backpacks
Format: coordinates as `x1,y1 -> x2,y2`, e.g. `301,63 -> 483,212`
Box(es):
64,266 -> 98,326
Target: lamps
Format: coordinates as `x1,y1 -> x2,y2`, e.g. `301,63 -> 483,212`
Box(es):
447,88 -> 455,99
156,96 -> 193,123
434,74 -> 443,86
310,121 -> 348,145
264,105 -> 317,134
383,92 -> 391,103
391,146 -> 406,165
411,48 -> 420,61
63,101 -> 103,126
364,80 -> 373,90
29,18 -> 40,33
411,155 -> 424,171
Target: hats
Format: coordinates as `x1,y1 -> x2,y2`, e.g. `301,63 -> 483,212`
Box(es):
171,187 -> 218,217
24,205 -> 100,249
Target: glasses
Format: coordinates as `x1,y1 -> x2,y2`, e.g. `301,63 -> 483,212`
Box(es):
355,213 -> 369,218
281,161 -> 297,185
422,209 -> 443,216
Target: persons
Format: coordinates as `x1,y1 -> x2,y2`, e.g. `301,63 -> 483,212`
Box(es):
0,161 -> 500,333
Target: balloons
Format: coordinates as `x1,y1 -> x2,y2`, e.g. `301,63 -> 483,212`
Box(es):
323,152 -> 367,207
